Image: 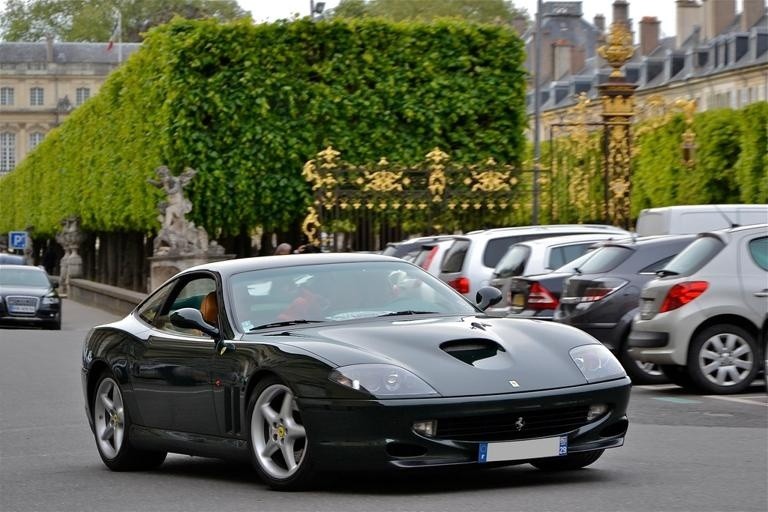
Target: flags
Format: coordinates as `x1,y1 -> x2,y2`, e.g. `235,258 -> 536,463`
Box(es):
107,15 -> 123,53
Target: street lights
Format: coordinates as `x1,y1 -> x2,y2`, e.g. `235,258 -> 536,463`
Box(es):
533,0 -> 584,235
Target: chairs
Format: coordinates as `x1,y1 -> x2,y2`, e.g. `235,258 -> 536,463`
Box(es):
201,290 -> 254,328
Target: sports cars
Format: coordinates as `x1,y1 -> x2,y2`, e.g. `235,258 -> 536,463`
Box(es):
81,252 -> 633,492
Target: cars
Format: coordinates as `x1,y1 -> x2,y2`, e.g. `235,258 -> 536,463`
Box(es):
350,202 -> 768,385
626,223 -> 768,395
0,252 -> 62,330
